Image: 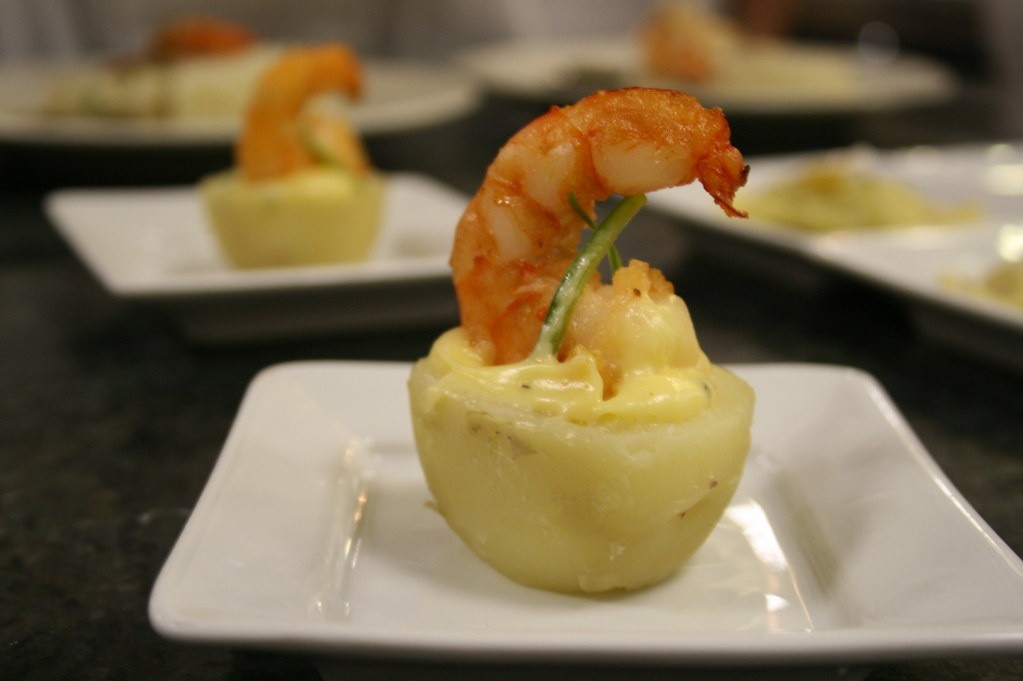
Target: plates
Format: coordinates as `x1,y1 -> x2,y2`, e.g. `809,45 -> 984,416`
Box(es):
0,45 -> 490,151
44,169 -> 475,349
610,141 -> 1023,371
462,36 -> 957,116
146,359 -> 1023,671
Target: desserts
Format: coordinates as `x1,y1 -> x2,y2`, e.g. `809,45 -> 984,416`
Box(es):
200,164 -> 382,268
409,261 -> 756,595
